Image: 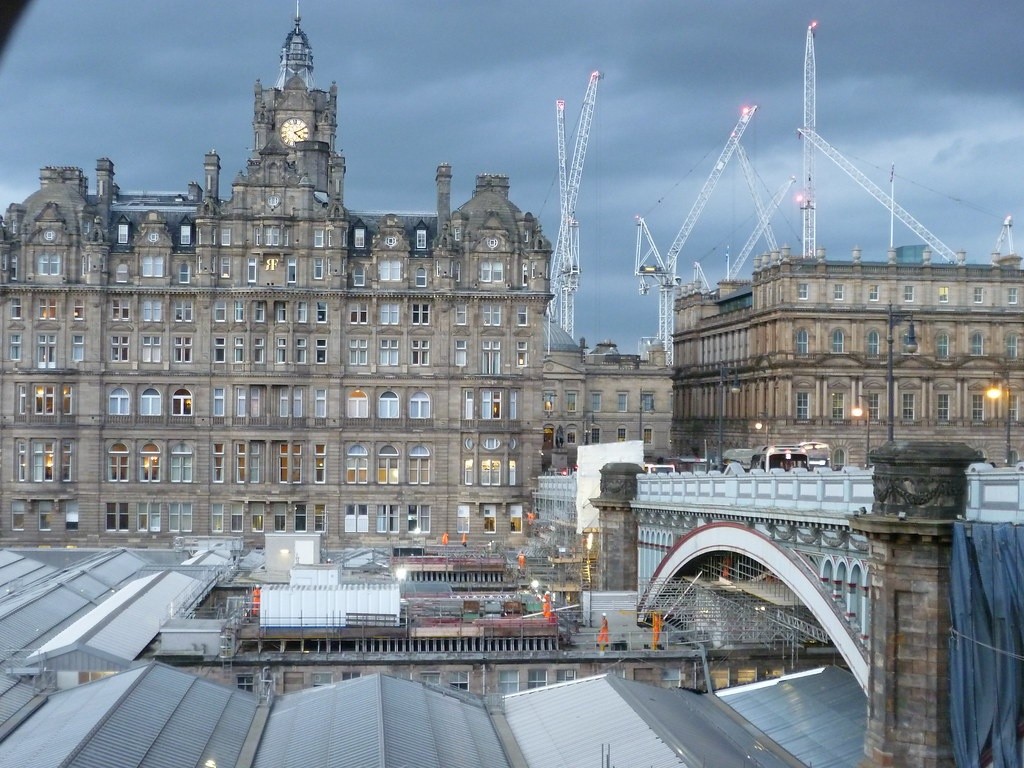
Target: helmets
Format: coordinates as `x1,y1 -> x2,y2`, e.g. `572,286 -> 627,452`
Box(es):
256,585 -> 260,588
546,591 -> 550,595
602,613 -> 606,617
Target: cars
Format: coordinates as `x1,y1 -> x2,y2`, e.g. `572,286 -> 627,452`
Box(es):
644,464 -> 675,475
659,459 -> 689,475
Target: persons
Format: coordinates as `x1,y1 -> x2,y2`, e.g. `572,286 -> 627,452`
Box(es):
442,530 -> 449,544
597,613 -> 609,647
527,512 -> 536,525
653,611 -> 663,641
462,533 -> 468,545
252,584 -> 260,617
517,551 -> 526,567
720,556 -> 730,577
541,591 -> 552,618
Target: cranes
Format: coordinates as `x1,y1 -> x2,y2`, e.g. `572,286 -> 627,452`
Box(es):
797,127 -> 1024,266
546,71 -> 605,337
795,20 -> 821,258
634,104 -> 756,363
722,141 -> 796,282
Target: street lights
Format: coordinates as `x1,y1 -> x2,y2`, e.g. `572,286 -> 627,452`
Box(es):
756,411 -> 769,446
987,383 -> 1011,466
585,427 -> 591,446
888,312 -> 918,441
853,394 -> 870,469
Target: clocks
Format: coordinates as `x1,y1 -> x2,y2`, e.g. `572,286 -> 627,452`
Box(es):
280,117 -> 311,148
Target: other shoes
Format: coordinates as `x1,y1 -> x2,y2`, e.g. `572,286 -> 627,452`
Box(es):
596,644 -> 599,647
604,644 -> 608,647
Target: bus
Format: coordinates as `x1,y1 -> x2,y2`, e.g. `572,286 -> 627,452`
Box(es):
721,445 -> 811,473
